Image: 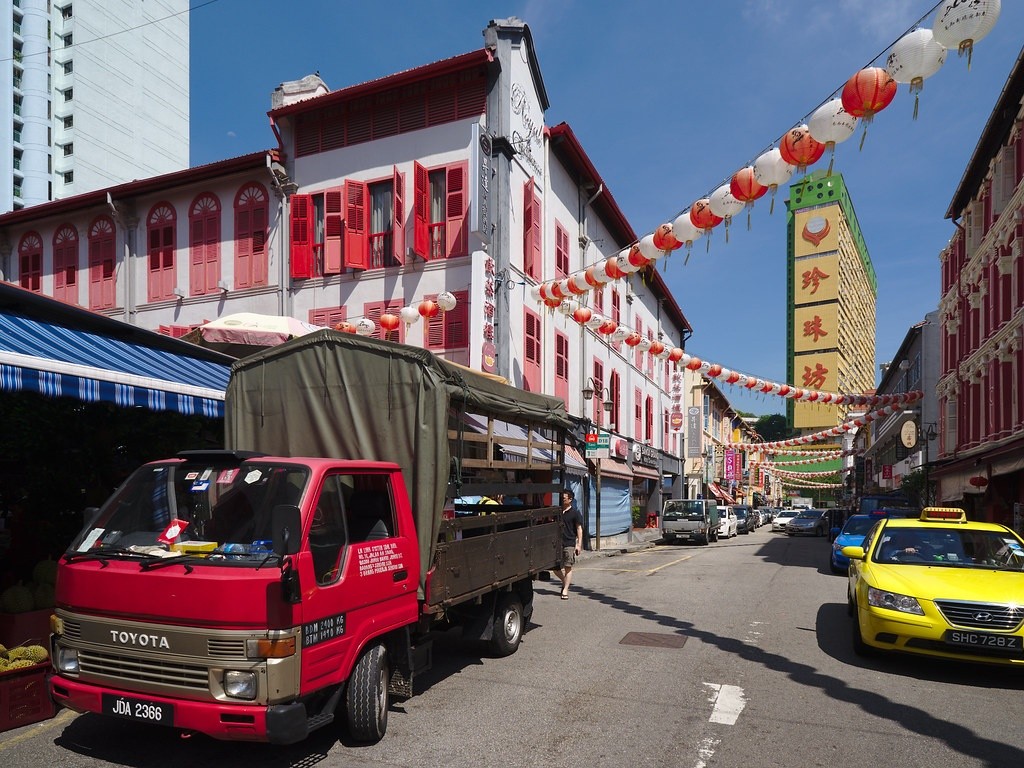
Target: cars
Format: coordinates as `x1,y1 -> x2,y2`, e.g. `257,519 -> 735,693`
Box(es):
840,506 -> 1024,668
829,509 -> 908,575
785,508 -> 840,537
753,505 -> 810,529
717,505 -> 738,539
771,510 -> 803,532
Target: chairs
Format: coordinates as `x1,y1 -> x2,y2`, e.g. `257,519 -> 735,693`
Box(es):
348,491 -> 394,543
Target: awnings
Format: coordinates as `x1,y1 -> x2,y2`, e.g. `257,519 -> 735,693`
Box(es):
0,313 -> 231,418
449,408 -> 660,481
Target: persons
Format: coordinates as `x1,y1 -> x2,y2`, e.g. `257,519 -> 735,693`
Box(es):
552,489 -> 584,600
905,548 -> 915,555
478,494 -> 503,516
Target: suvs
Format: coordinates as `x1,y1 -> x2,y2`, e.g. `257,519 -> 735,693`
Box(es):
732,504 -> 756,535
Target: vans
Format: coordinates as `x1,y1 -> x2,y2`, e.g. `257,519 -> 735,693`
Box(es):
856,495 -> 921,518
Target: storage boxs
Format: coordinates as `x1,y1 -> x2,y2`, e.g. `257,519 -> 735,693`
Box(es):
0,657 -> 56,732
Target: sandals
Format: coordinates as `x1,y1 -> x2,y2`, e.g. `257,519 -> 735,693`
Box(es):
561,594 -> 569,600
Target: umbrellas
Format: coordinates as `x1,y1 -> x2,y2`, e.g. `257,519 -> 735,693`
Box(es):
179,313 -> 321,346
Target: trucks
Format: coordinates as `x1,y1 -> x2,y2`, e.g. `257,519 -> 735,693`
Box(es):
655,498 -> 723,545
46,327 -> 575,749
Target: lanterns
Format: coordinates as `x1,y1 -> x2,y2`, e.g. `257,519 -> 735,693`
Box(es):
969,471 -> 987,491
530,0 -> 1002,309
559,300 -> 923,405
725,403 -> 898,488
335,292 -> 456,336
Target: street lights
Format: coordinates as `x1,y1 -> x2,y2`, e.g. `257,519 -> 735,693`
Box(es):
874,459 -> 881,487
581,376 -> 615,552
918,424 -> 938,507
701,445 -> 714,499
